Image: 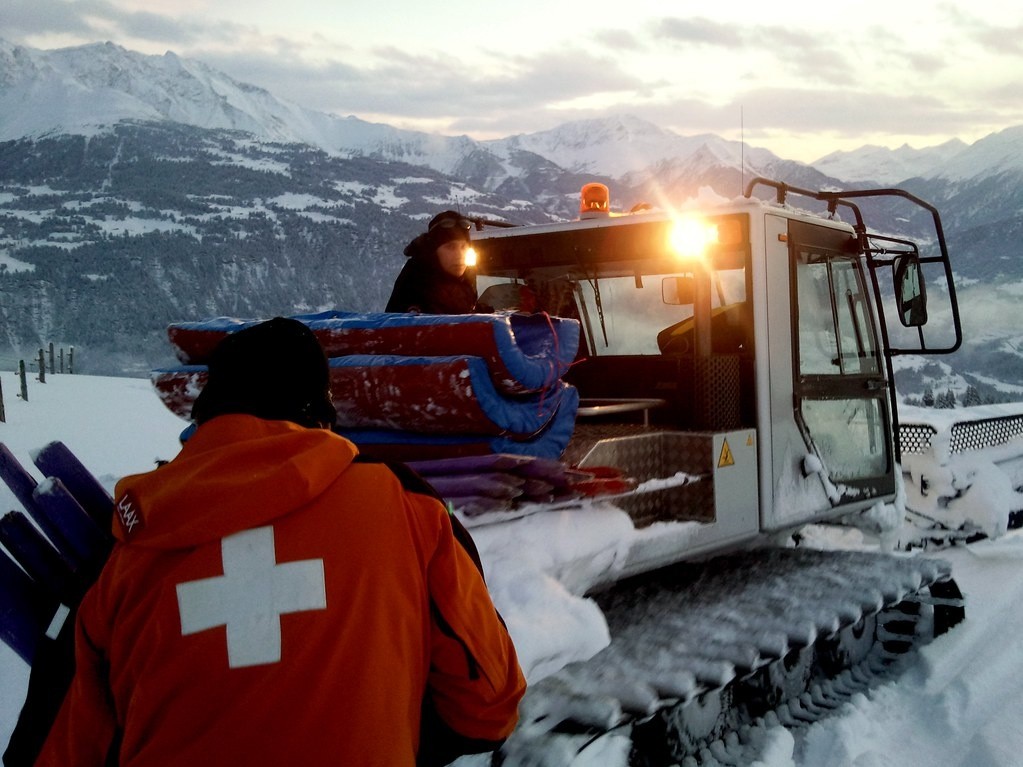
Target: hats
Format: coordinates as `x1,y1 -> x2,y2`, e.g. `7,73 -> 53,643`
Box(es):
191,315 -> 331,432
428,211 -> 472,250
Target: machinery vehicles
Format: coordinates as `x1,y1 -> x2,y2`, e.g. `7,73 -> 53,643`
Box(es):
435,176 -> 967,767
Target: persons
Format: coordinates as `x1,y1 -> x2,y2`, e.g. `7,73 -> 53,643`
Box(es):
0,317 -> 528,767
385,210 -> 495,314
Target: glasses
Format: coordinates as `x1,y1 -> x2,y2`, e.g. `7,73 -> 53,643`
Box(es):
431,218 -> 471,232
